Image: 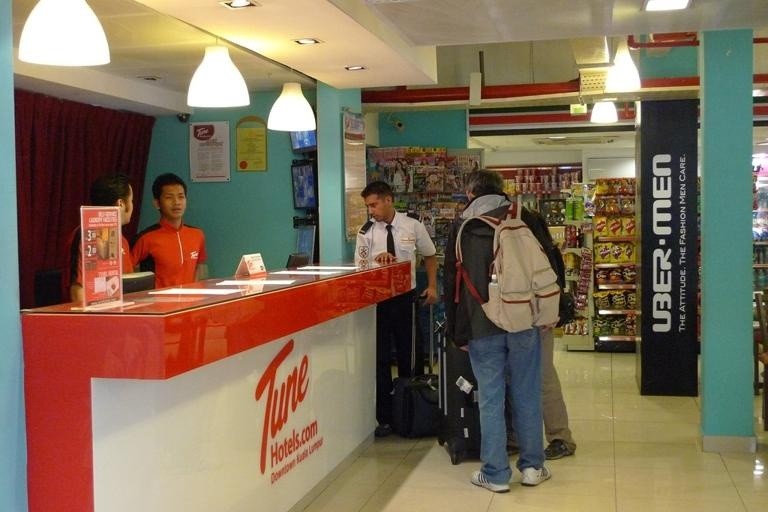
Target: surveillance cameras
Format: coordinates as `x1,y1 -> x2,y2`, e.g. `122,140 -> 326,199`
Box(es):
177,113 -> 190,122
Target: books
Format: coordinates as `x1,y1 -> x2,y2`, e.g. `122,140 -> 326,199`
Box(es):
365,146 -> 480,265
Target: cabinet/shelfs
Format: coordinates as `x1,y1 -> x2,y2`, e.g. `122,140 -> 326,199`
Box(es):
753,175 -> 768,348
485,165 -> 640,354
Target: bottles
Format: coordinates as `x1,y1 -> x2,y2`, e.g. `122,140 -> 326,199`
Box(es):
752,269 -> 758,289
753,246 -> 763,264
757,270 -> 766,290
489,275 -> 500,288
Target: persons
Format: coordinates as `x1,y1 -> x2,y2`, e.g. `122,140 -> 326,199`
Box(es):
354,181 -> 438,438
129,173 -> 209,288
68,174 -> 134,305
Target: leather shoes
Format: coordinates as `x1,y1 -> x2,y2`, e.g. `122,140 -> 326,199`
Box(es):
375,423 -> 392,437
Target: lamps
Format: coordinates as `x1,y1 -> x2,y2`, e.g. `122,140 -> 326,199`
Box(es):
587,99 -> 619,126
604,37 -> 638,91
18,0 -> 110,67
187,37 -> 250,108
264,66 -> 316,131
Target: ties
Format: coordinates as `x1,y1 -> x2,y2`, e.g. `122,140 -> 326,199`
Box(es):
386,225 -> 395,256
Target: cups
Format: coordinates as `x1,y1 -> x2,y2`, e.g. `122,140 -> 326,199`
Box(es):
515,166 -> 564,192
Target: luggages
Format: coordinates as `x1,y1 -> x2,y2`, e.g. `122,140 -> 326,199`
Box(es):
437,333 -> 481,464
388,295 -> 439,438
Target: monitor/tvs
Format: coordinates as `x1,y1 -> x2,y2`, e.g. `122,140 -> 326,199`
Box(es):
286,253 -> 308,268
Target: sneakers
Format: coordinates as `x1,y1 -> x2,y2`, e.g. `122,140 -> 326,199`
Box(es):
544,438 -> 570,460
471,469 -> 510,492
506,444 -> 520,455
522,463 -> 550,485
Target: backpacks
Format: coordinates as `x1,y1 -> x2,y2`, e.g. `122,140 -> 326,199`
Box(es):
454,200 -> 562,333
